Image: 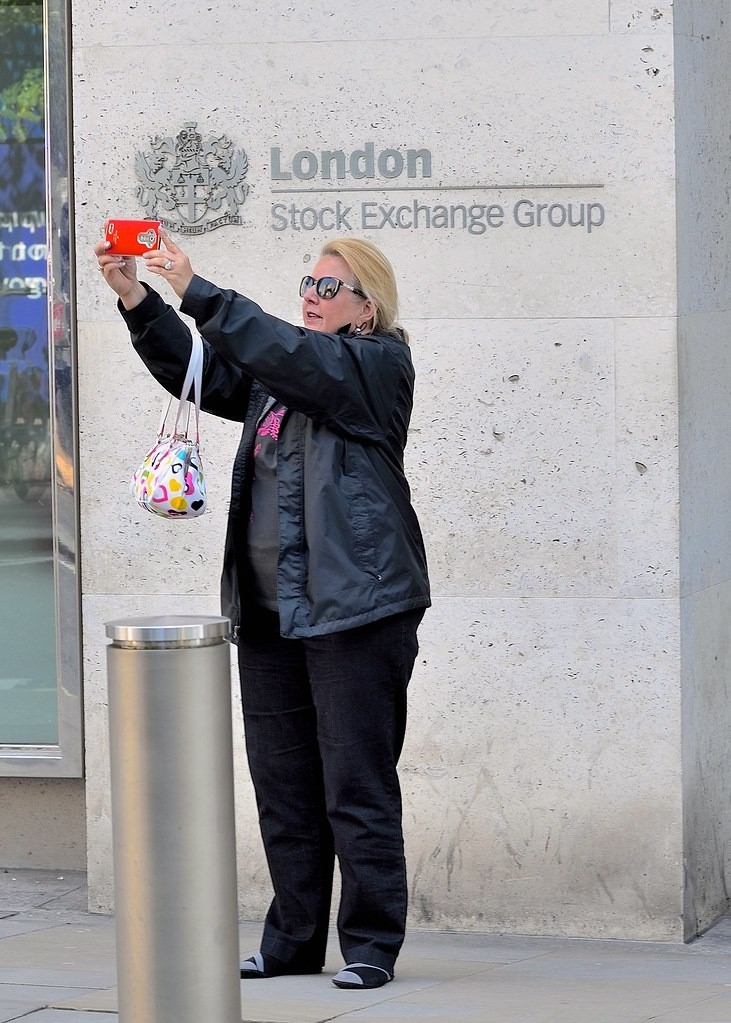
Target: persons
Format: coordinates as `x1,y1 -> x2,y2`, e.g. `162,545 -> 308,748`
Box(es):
94,228 -> 431,989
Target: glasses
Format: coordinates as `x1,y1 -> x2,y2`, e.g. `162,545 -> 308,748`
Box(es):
299,275 -> 368,301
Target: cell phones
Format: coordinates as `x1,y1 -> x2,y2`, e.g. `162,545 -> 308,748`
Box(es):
104,219 -> 163,257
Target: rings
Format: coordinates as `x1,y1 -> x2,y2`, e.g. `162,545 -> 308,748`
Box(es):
165,261 -> 171,270
97,261 -> 104,271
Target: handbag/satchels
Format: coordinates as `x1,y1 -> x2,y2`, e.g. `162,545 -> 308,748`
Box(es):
132,334 -> 207,520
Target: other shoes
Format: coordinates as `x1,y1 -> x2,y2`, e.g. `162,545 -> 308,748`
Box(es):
332,962 -> 392,989
239,952 -> 322,978
